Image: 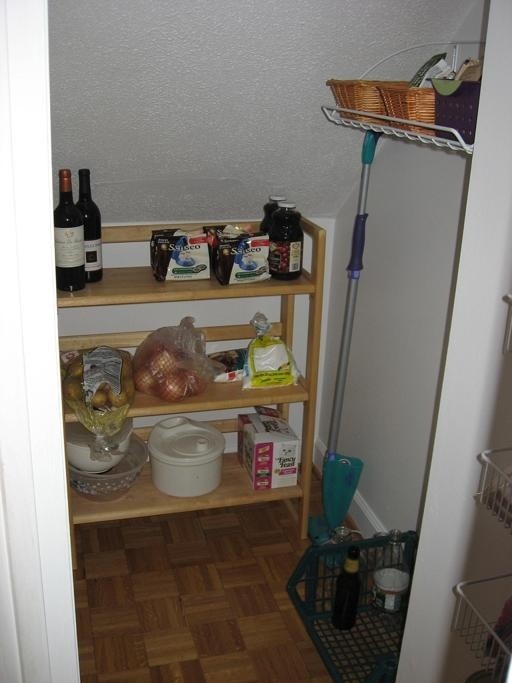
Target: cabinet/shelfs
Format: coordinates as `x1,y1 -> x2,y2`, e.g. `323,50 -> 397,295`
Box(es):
55,219 -> 324,571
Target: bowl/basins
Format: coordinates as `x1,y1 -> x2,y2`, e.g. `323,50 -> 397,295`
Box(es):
65,417 -> 135,474
68,432 -> 149,502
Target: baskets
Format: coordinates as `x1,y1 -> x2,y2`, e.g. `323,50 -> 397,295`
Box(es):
327,79 -> 481,144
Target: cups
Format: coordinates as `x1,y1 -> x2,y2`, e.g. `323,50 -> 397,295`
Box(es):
371,567 -> 411,615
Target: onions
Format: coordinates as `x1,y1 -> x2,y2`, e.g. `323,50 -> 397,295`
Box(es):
135,339 -> 208,402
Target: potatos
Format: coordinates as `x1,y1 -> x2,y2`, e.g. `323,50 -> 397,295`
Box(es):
63,354 -> 135,409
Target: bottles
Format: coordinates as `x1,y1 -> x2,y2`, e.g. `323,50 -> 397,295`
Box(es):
74,167 -> 106,284
330,546 -> 361,629
259,193 -> 304,281
54,168 -> 86,292
